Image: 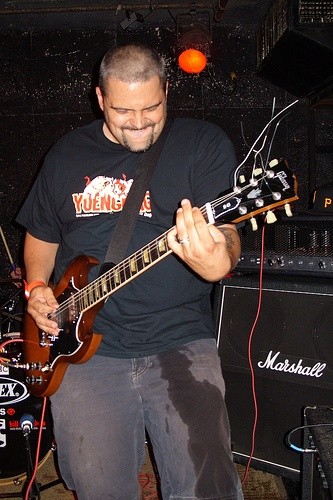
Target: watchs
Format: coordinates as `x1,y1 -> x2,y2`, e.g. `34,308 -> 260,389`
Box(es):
25,281 -> 47,299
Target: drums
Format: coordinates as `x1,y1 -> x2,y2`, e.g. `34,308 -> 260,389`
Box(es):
1,335 -> 58,484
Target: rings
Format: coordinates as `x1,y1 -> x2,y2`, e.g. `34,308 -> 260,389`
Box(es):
179,236 -> 190,245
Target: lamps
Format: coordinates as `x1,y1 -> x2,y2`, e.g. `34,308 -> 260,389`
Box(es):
176,11 -> 213,74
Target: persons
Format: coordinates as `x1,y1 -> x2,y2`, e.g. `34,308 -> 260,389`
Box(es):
17,45 -> 243,500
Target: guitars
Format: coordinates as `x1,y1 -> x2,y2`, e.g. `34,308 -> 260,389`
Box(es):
22,157 -> 298,398
